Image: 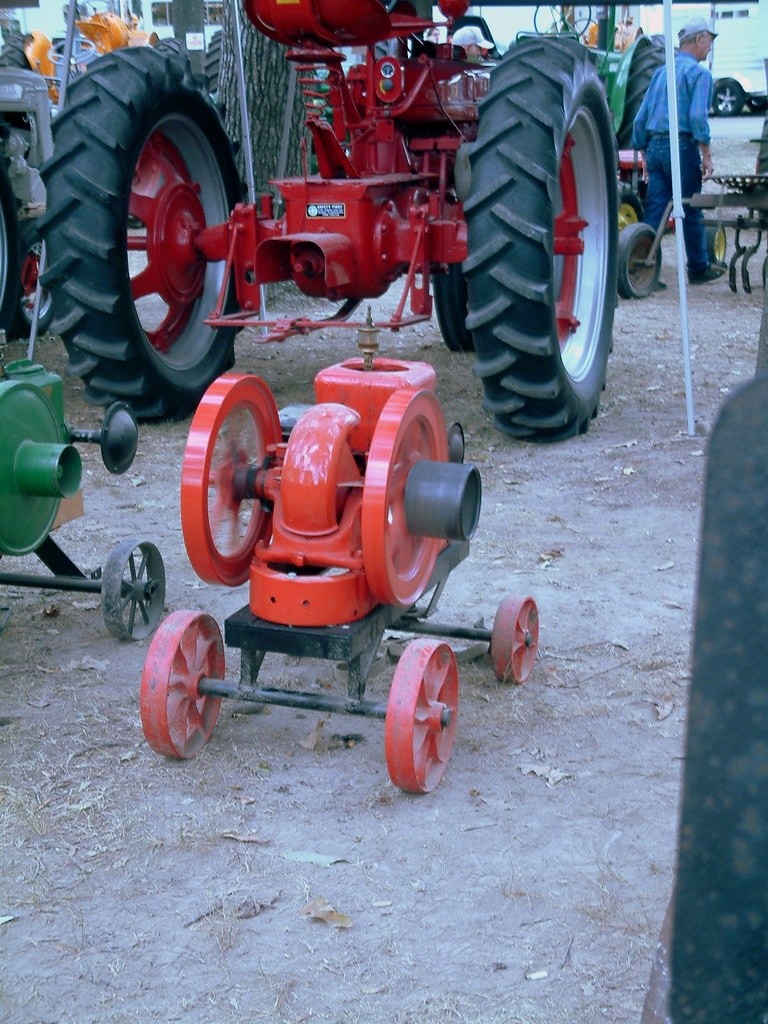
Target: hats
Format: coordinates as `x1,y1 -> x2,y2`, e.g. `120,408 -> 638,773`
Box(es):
452,26 -> 494,49
677,17 -> 719,41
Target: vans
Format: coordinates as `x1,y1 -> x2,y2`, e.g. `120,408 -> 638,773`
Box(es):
640,0 -> 768,118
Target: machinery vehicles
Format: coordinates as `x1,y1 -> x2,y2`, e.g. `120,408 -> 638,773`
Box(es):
0,0 -> 728,444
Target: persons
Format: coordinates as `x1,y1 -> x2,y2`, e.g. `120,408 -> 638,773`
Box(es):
633,17 -> 727,290
453,26 -> 494,56
429,28 -> 440,42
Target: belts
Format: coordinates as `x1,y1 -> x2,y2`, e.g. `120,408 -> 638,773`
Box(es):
651,133 -> 690,139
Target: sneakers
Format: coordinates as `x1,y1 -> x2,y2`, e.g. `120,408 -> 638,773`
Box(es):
688,260 -> 728,284
653,281 -> 667,291
